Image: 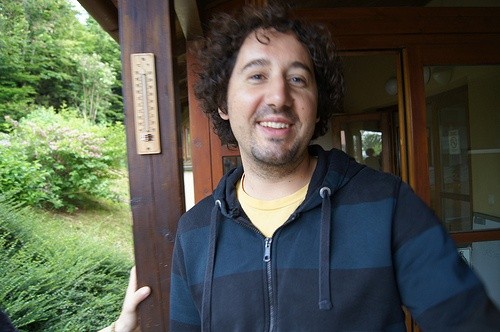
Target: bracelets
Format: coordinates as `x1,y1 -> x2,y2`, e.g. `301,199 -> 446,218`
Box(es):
110,321 -> 117,332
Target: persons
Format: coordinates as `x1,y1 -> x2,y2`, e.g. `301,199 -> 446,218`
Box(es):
361,147 -> 383,171
163,0 -> 500,332
0,262 -> 153,332
